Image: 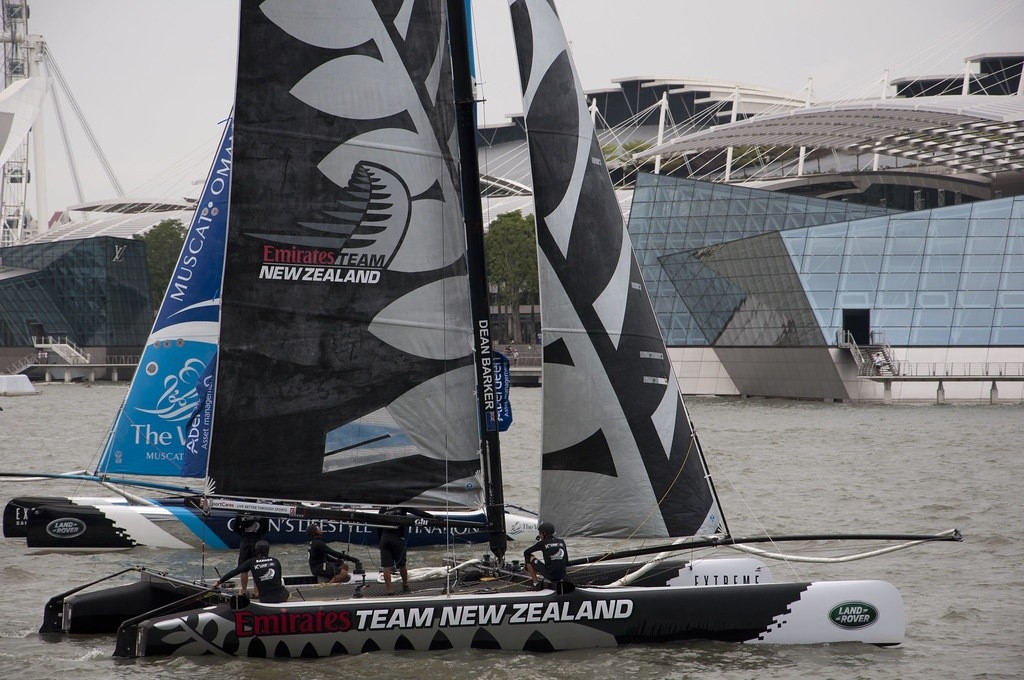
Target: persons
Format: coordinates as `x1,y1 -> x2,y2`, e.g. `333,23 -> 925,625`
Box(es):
523,521 -> 570,592
874,353 -> 884,377
502,330 -> 543,365
37,350 -> 49,365
231,498 -> 269,598
374,505 -> 411,596
306,523 -> 359,584
208,540 -> 291,605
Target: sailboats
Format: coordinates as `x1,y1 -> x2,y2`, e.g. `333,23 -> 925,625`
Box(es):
3,0 -> 971,664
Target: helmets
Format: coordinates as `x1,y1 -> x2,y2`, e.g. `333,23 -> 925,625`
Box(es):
255,541 -> 270,551
538,523 -> 555,533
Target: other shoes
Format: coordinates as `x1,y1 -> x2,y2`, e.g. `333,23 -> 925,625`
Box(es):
402,585 -> 409,591
526,582 -> 540,591
388,593 -> 394,596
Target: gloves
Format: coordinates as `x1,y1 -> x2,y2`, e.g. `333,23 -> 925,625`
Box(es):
348,557 -> 359,563
